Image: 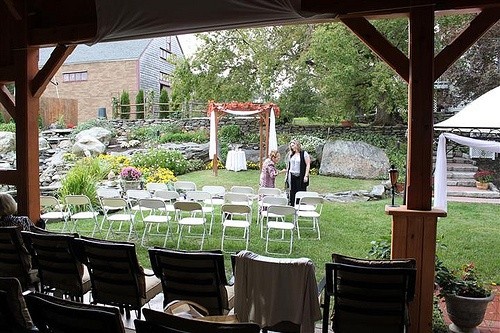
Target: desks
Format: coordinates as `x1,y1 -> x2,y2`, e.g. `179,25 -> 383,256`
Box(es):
226,151 -> 247,172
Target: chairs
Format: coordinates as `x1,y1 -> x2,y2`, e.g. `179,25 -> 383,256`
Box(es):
40,181 -> 323,255
0,225 -> 415,333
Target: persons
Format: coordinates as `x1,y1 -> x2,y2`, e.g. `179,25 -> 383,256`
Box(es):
259,150 -> 288,219
0,192 -> 35,272
284,140 -> 311,217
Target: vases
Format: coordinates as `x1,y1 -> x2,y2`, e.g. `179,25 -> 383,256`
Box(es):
475,182 -> 489,190
123,180 -> 141,192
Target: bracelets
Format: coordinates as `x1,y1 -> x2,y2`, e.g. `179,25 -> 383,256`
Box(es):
305,175 -> 309,177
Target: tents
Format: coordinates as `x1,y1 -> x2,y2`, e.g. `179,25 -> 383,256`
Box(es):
433,85 -> 500,197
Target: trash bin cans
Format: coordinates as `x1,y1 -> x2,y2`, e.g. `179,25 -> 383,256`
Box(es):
98,107 -> 106,120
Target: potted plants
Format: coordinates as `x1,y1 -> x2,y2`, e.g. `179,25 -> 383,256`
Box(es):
441,279 -> 494,333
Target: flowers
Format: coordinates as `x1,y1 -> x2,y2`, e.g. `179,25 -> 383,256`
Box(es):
473,171 -> 498,183
120,167 -> 141,181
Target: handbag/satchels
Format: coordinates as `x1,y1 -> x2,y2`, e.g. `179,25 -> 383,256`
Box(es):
163,299 -> 239,325
